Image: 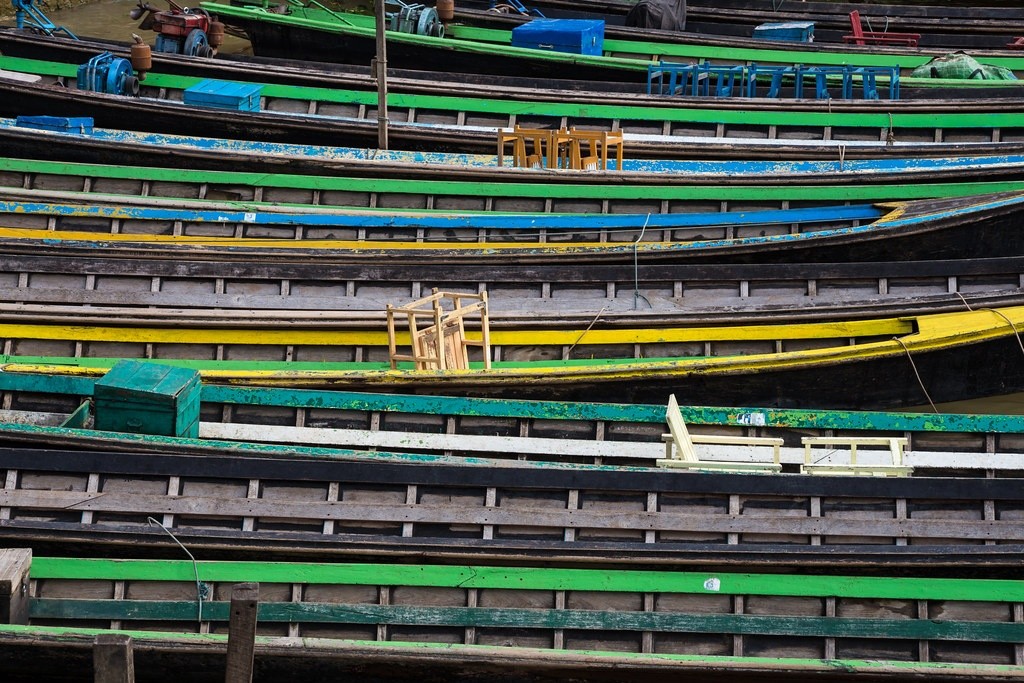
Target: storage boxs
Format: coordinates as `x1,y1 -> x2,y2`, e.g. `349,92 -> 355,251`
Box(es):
512,17 -> 605,56
16,116 -> 95,136
95,360 -> 201,439
752,22 -> 816,43
184,79 -> 264,112
0,548 -> 32,627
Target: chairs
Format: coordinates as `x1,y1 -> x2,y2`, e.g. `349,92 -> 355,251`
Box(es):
843,10 -> 921,47
656,394 -> 784,473
